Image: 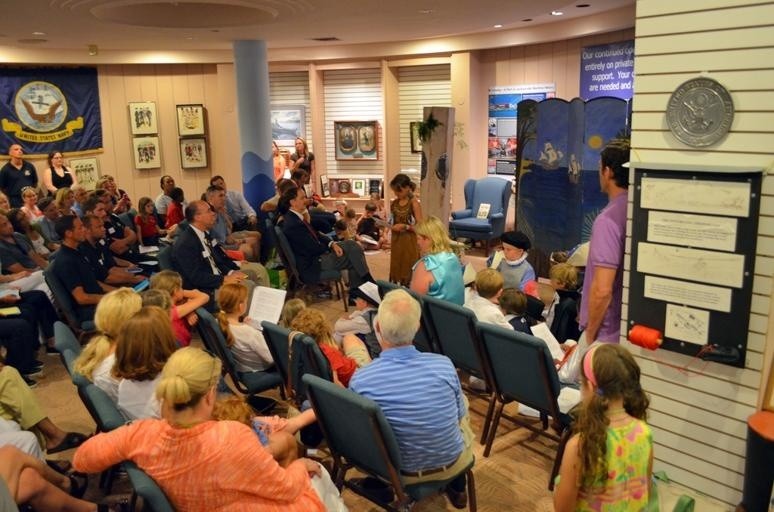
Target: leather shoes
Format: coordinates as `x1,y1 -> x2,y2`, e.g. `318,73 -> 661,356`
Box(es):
445,484 -> 467,509
349,478 -> 394,502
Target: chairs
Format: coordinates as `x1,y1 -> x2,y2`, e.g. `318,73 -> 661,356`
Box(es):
260,322 -> 341,481
130,208 -> 138,219
422,297 -> 495,444
274,227 -> 348,312
42,248 -> 174,512
302,374 -> 477,511
377,282 -> 430,355
196,307 -> 283,393
476,322 -> 582,492
117,213 -> 134,230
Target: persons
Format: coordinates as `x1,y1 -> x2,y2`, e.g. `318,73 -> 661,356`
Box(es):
0,443 -> 145,511
553,341 -> 653,512
348,288 -> 475,509
72,345 -> 352,512
576,141 -> 630,358
0,136 -> 391,422
0,358 -> 94,456
384,174 -> 575,421
0,420 -> 71,473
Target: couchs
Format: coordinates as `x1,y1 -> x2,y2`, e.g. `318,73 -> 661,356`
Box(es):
450,176 -> 512,255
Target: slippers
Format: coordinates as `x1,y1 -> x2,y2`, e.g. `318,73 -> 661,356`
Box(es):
47,433 -> 93,454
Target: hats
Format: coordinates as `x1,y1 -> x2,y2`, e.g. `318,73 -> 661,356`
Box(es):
501,231 -> 530,249
351,281 -> 384,307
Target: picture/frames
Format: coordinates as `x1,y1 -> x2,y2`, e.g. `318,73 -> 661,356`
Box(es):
338,180 -> 350,193
370,180 -> 381,196
409,122 -> 422,153
353,180 -> 365,195
180,138 -> 207,169
176,104 -> 204,135
321,175 -> 331,197
270,104 -> 306,146
